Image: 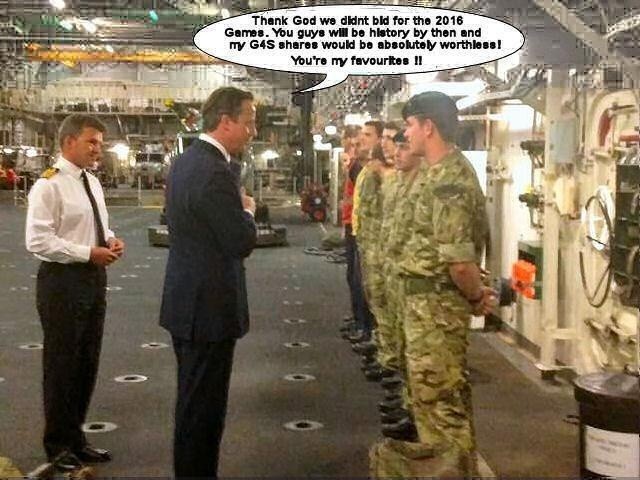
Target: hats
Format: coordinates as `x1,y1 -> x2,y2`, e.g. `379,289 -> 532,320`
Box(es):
392,128 -> 407,143
402,91 -> 458,118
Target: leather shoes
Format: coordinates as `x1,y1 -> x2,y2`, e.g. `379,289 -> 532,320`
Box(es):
75,442 -> 112,462
48,451 -> 82,471
341,316 -> 418,441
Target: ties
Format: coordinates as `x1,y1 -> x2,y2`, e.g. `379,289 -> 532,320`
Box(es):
81,170 -> 107,247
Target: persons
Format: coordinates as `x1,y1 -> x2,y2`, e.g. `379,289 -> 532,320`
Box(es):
158,87 -> 258,480
25,115 -> 125,471
340,91 -> 500,480
